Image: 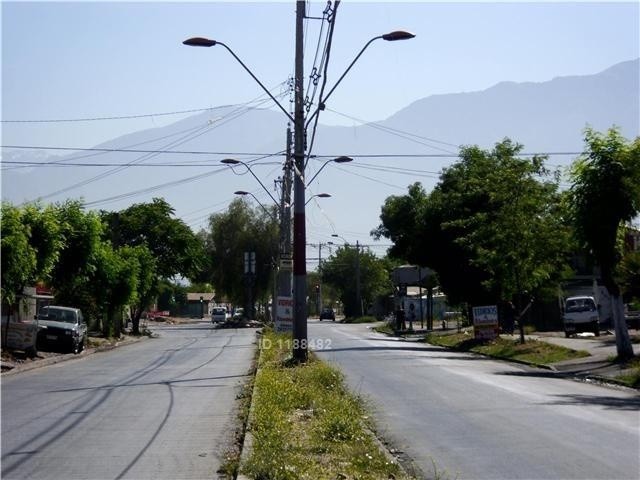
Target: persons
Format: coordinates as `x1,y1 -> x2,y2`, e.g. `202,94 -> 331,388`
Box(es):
395,304 -> 406,330
408,303 -> 416,330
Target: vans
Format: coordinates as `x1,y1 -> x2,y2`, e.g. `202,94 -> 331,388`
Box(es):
563,296 -> 601,338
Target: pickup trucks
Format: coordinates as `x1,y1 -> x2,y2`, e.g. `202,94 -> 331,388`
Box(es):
34,305 -> 87,354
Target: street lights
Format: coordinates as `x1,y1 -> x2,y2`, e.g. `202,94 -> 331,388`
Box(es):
182,31 -> 416,365
